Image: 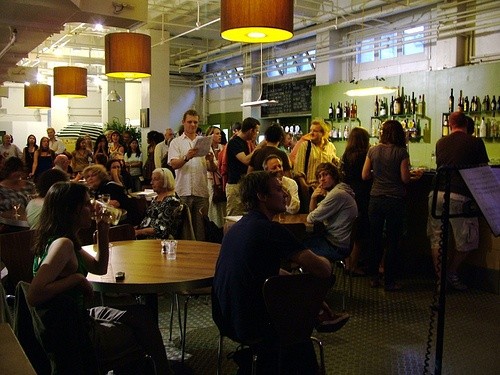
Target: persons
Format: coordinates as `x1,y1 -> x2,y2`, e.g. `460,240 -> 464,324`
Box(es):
0,110 -> 499,375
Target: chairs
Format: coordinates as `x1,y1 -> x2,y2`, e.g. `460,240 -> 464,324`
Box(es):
216,273 -> 331,375
199,206 -> 223,243
93,224 -> 136,243
0,230 -> 38,286
330,212 -> 362,312
169,286 -> 213,364
18,280 -> 157,375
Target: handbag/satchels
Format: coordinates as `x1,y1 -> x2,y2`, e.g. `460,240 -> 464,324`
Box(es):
292,177 -> 303,200
213,184 -> 227,203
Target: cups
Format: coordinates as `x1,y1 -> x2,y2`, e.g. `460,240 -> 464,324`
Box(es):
98,195 -> 104,201
89,198 -> 122,226
102,194 -> 110,204
108,243 -> 112,265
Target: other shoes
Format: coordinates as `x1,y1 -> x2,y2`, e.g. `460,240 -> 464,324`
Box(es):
316,312 -> 349,332
369,275 -> 379,287
384,283 -> 403,290
448,271 -> 468,291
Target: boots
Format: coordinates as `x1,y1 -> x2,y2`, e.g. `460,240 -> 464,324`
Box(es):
346,263 -> 365,277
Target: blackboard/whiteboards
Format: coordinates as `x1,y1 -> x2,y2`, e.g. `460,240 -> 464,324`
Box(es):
261,74 -> 316,117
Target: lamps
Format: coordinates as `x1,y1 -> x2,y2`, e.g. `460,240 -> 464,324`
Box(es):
105,32 -> 151,78
24,82 -> 51,109
54,67 -> 87,98
239,44 -> 278,106
106,89 -> 123,102
220,0 -> 294,43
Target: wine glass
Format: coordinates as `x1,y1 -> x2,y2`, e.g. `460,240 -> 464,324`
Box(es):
12,200 -> 21,217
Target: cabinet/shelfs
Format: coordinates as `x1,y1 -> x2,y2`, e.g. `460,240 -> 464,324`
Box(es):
324,118 -> 360,142
441,111 -> 500,139
370,114 -> 430,140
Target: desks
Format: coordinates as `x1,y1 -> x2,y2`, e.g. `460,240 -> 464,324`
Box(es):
0,210 -> 30,228
223,211 -> 314,235
37,240 -> 221,293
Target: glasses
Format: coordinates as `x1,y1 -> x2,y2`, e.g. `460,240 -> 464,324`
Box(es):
112,166 -> 121,168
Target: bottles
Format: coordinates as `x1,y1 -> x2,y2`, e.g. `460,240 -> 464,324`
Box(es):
329,125 -> 349,141
456,90 -> 500,111
449,88 -> 454,112
329,99 -> 357,121
374,86 -> 425,116
431,153 -> 437,169
442,112 -> 500,137
372,119 -> 421,139
92,230 -> 98,251
161,239 -> 177,259
27,177 -> 29,180
